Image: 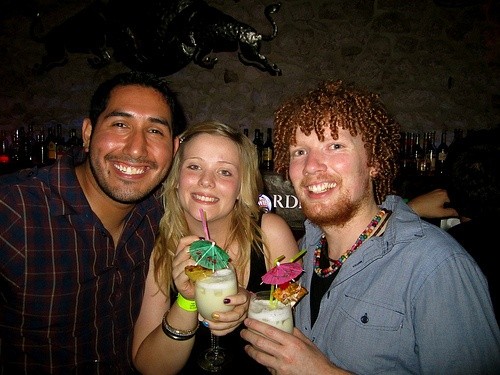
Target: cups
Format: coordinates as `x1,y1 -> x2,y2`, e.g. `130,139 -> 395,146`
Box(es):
194,262 -> 237,324
248,290 -> 293,345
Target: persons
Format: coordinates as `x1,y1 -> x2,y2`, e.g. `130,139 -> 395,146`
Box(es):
0,71 -> 458,375
240,80 -> 500,375
132,119 -> 303,375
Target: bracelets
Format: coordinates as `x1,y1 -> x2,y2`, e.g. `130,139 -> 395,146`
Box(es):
162,311 -> 198,341
177,293 -> 198,311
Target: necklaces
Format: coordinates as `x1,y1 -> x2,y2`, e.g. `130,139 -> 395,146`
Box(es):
313,209 -> 391,277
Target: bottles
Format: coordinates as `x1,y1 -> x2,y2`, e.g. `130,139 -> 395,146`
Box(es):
399,125 -> 500,185
0,123 -> 87,173
244,127 -> 274,172
